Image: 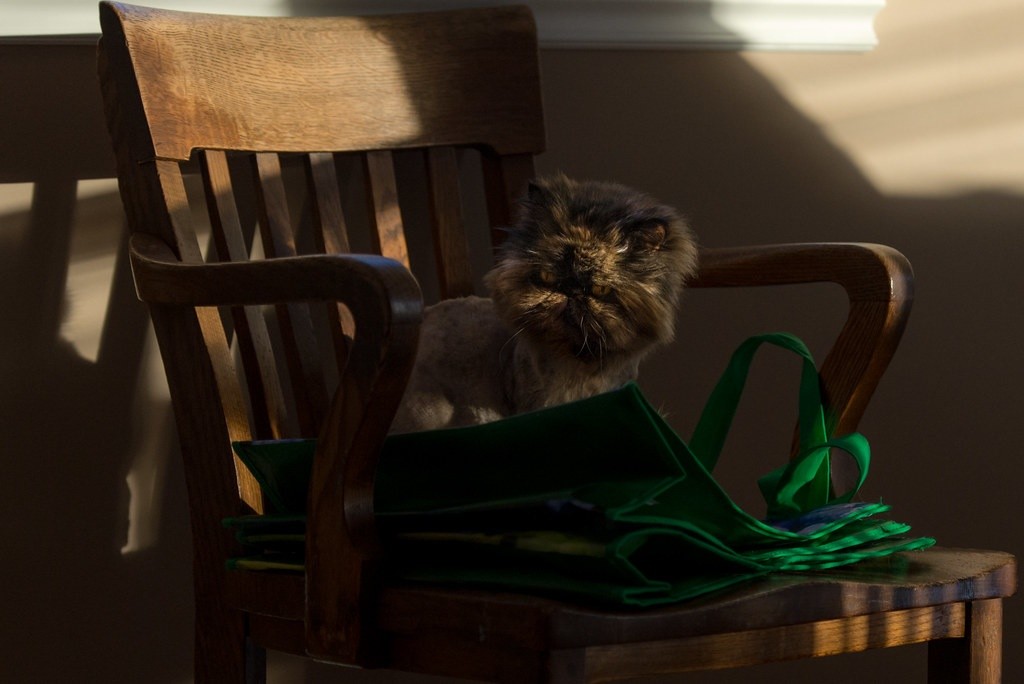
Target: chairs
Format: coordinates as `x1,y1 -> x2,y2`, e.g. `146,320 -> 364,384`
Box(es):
97,0 -> 1019,684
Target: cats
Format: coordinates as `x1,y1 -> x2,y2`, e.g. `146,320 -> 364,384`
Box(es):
384,171 -> 701,436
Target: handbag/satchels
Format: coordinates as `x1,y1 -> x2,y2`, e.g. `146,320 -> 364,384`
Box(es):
220,331 -> 937,614
